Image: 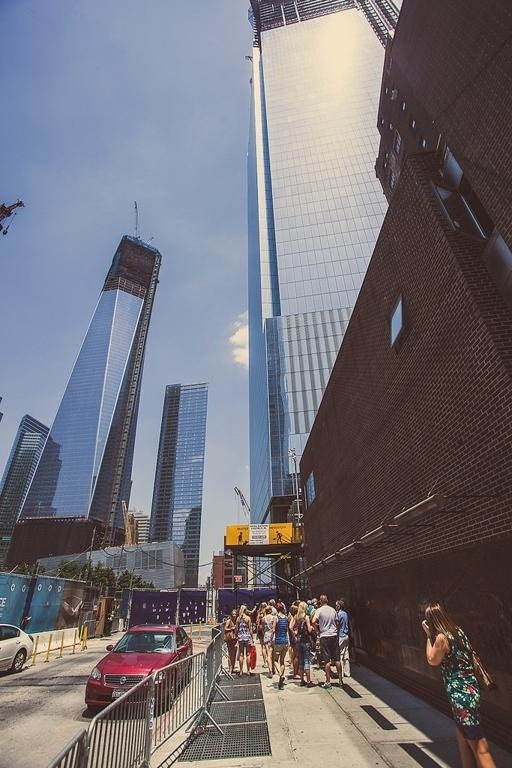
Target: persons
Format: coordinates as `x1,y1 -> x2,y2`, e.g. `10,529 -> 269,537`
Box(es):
223,593 -> 359,689
422,600 -> 497,768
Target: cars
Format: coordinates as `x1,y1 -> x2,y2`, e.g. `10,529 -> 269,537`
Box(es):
62,595 -> 82,618
0,623 -> 35,675
86,624 -> 193,715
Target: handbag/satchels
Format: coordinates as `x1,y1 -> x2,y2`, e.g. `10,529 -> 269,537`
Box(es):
250,644 -> 256,669
223,630 -> 236,641
469,652 -> 497,692
299,621 -> 308,634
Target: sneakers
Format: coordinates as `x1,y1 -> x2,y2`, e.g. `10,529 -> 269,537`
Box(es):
279,675 -> 285,687
266,672 -> 272,678
320,683 -> 331,688
299,680 -> 307,686
307,681 -> 315,687
